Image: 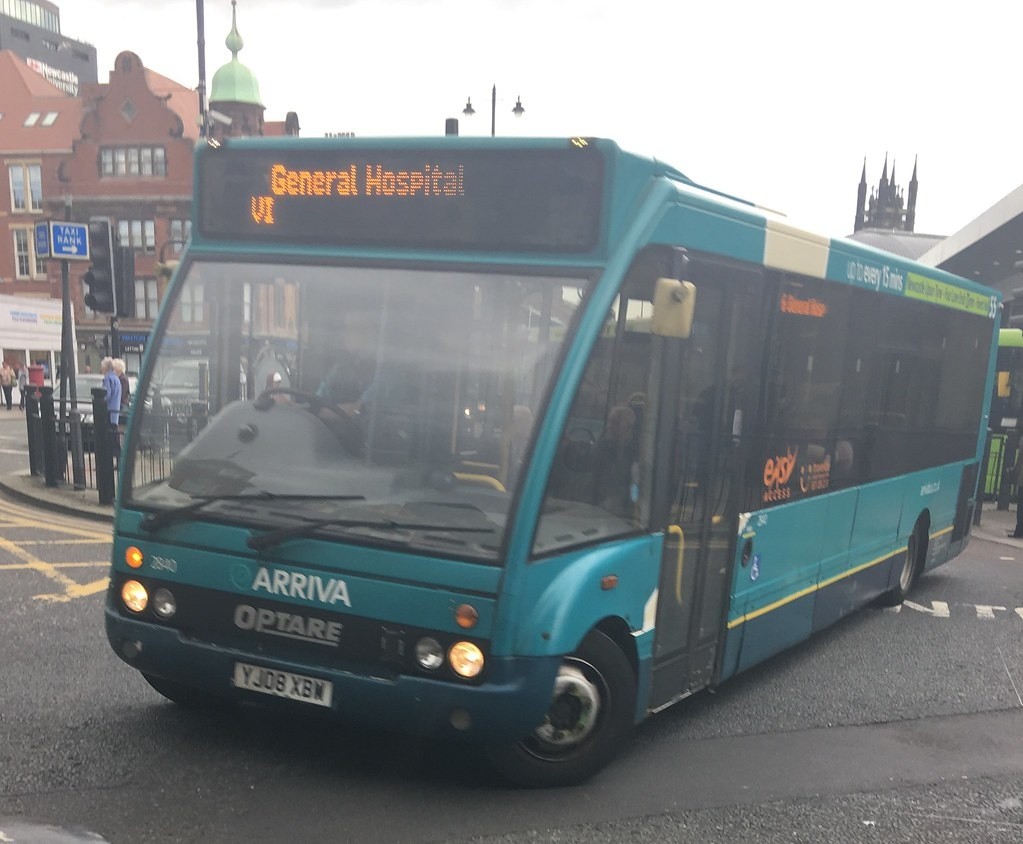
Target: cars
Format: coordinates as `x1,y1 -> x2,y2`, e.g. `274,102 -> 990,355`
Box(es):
37,372 -> 174,453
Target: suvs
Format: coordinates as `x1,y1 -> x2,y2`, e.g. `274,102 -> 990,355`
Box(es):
158,354 -> 248,419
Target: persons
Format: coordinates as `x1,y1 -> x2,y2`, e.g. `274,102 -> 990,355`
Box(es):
93,356 -> 122,471
0,361 -> 15,411
270,304 -> 412,468
502,353 -> 862,505
112,358 -> 130,470
18,363 -> 30,412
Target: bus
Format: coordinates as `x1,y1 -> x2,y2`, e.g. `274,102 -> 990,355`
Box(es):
981,324 -> 1022,499
100,130 -> 1005,787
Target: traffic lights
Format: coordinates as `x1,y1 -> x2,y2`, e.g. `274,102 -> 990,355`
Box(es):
83,212 -> 119,319
